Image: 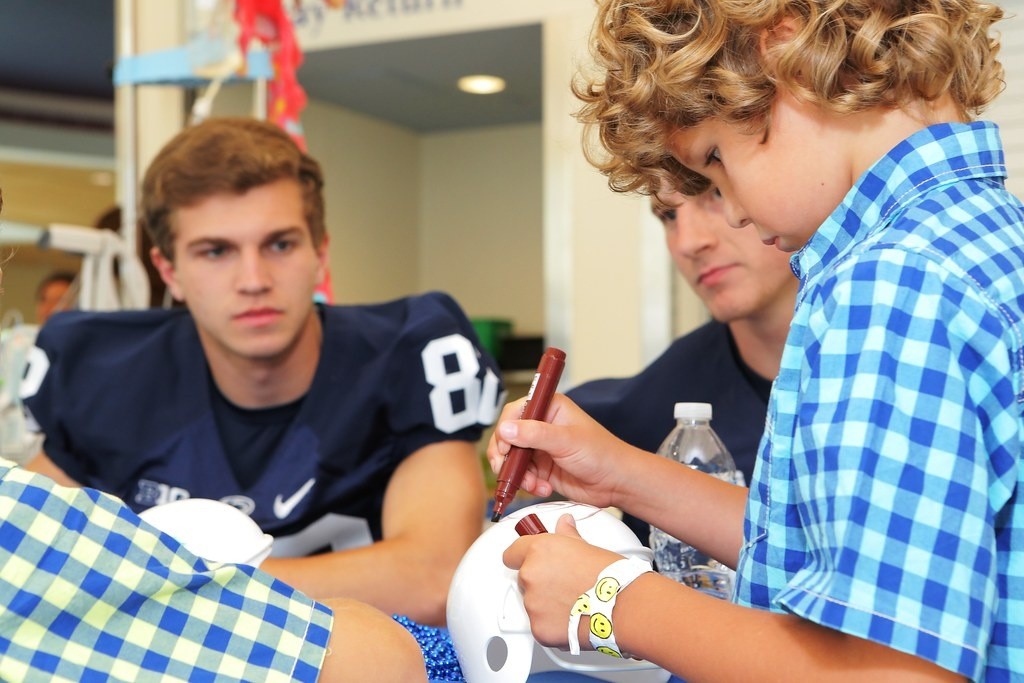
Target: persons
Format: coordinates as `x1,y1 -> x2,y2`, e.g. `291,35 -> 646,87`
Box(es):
0,0 -> 1024,683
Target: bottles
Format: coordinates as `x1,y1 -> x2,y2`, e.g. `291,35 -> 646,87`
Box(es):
649,403 -> 746,601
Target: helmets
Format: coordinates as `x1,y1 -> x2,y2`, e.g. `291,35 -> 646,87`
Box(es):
135,498 -> 274,570
445,501 -> 672,683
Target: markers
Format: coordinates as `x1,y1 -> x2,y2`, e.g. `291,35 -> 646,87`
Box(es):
491,347 -> 566,522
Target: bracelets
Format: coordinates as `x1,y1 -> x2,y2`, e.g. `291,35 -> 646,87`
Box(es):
568,555 -> 652,660
